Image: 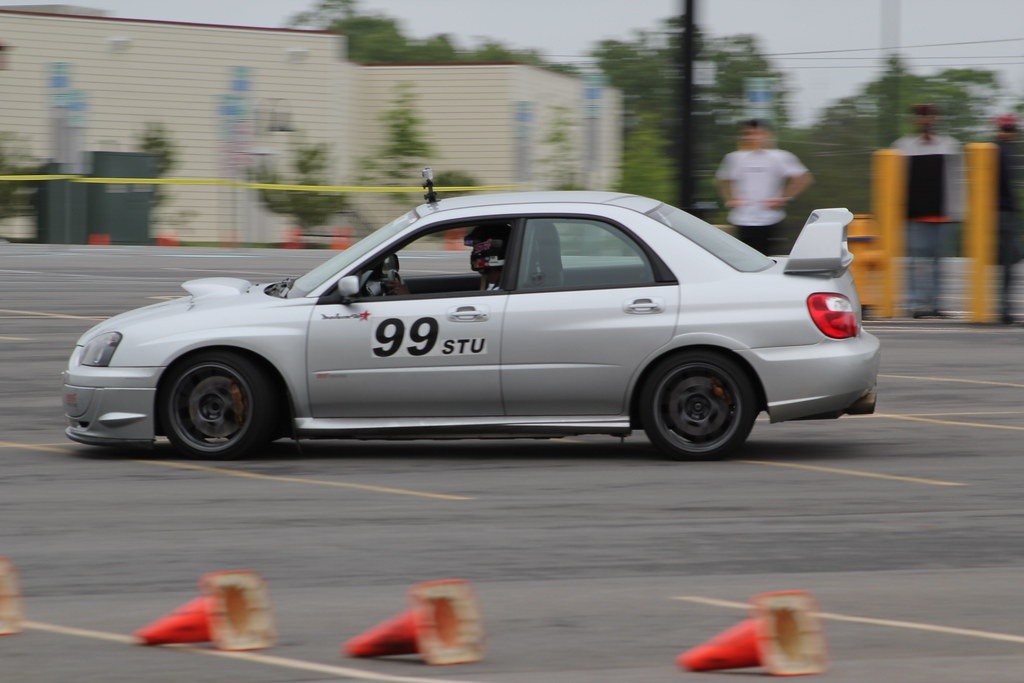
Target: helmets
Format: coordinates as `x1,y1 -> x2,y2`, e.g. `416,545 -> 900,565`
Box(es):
464,222 -> 512,271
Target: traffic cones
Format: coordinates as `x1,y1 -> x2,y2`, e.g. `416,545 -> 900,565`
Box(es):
676,588 -> 831,677
132,568 -> 278,650
340,577 -> 485,666
0,555 -> 24,636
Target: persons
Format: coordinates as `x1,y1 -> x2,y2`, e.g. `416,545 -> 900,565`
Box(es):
889,103 -> 970,319
716,119 -> 816,257
990,110 -> 1024,329
378,219 -> 517,298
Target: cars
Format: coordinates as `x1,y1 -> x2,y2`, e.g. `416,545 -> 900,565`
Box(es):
60,189 -> 881,461
